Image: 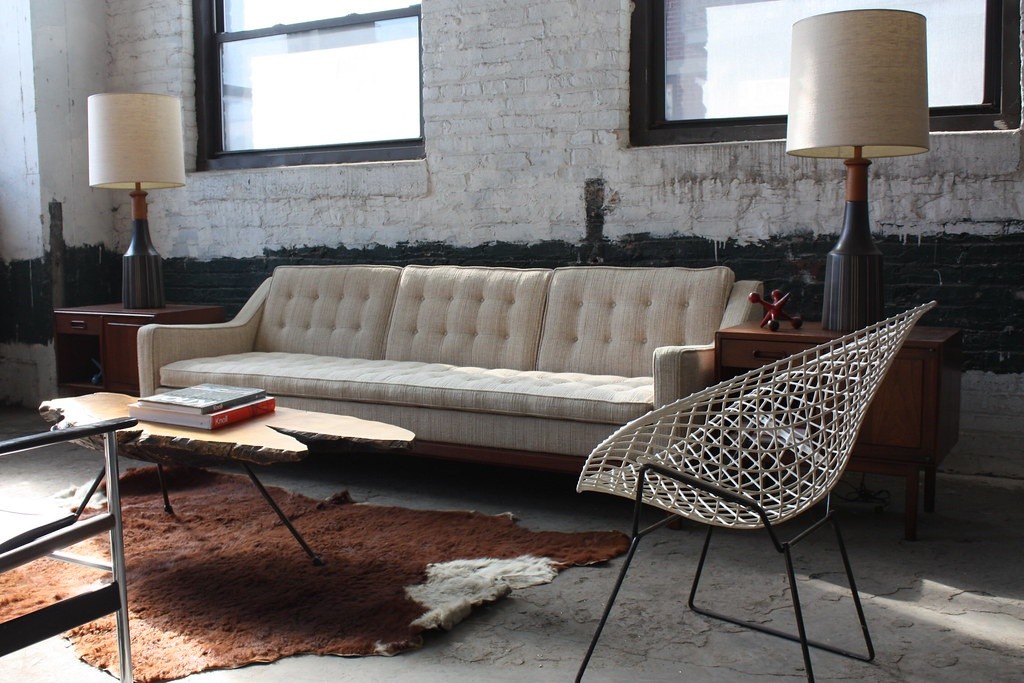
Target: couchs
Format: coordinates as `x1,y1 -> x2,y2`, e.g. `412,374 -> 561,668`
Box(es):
136,264 -> 765,530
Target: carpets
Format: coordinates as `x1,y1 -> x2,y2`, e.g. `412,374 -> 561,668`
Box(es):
0,463 -> 631,683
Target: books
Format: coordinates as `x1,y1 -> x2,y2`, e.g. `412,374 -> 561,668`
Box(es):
129,383 -> 275,430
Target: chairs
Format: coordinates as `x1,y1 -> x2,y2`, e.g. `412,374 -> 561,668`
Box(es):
575,298 -> 936,683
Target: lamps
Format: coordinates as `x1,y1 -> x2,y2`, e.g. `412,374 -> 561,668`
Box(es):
87,92 -> 186,309
783,7 -> 932,335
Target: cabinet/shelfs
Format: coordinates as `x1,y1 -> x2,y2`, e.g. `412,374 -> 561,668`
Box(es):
53,303 -> 224,398
715,320 -> 962,541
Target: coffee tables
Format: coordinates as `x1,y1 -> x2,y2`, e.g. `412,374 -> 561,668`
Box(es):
38,393 -> 416,566
